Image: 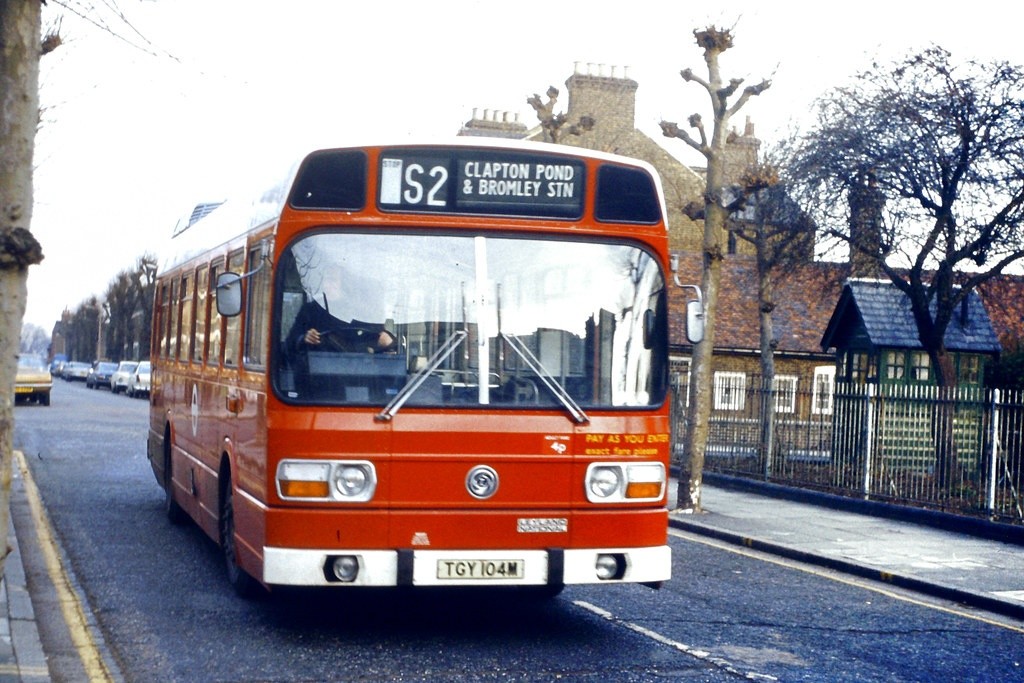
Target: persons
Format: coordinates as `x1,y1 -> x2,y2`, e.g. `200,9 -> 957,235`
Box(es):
285,257 -> 404,358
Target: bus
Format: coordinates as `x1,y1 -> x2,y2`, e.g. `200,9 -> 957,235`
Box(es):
147,135 -> 707,600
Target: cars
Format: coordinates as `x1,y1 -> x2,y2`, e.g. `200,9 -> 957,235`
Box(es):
87,361 -> 120,390
49,352 -> 101,385
12,354 -> 53,406
127,360 -> 151,400
108,360 -> 140,394
